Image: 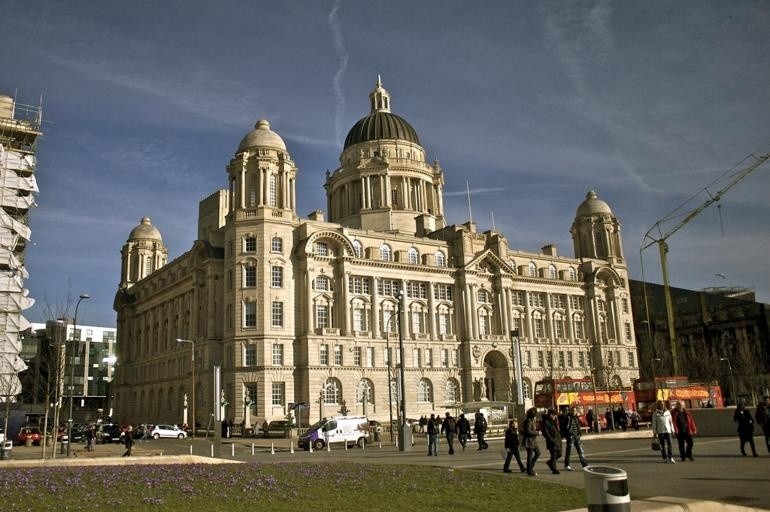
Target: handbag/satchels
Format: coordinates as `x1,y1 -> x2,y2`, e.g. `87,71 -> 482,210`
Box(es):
650,436 -> 661,451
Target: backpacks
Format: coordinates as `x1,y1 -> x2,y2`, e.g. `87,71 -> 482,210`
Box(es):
430,420 -> 439,435
448,418 -> 456,431
481,418 -> 487,432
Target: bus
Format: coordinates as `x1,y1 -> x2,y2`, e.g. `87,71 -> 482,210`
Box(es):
535,377 -> 638,433
633,377 -> 724,424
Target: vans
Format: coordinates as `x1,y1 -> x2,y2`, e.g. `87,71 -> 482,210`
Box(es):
298,415 -> 373,452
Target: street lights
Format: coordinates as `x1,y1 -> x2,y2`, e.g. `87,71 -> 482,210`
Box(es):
176,338 -> 196,438
651,358 -> 660,387
66,292 -> 90,458
396,289 -> 407,426
721,357 -> 737,407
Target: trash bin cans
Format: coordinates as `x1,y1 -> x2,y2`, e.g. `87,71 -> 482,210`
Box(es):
0,439 -> 12,459
582,465 -> 631,512
61,435 -> 69,454
373,426 -> 382,442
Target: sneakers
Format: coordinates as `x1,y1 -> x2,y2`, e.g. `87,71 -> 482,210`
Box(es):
427,453 -> 437,456
665,457 -> 676,464
681,457 -> 694,461
502,458 -> 588,477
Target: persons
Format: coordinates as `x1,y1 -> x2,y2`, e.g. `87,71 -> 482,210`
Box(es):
419,408 -> 488,456
122,425 -> 136,457
734,402 -> 759,457
252,421 -> 259,438
671,400 -> 696,461
755,396 -> 770,453
87,426 -> 96,452
707,401 -> 712,407
503,406 -> 595,476
262,420 -> 269,438
605,406 -> 642,431
651,400 -> 676,464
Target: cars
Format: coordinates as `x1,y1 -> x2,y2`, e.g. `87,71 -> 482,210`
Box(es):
0,419 -> 188,450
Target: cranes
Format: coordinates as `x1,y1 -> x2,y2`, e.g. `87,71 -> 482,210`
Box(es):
639,144 -> 769,376
